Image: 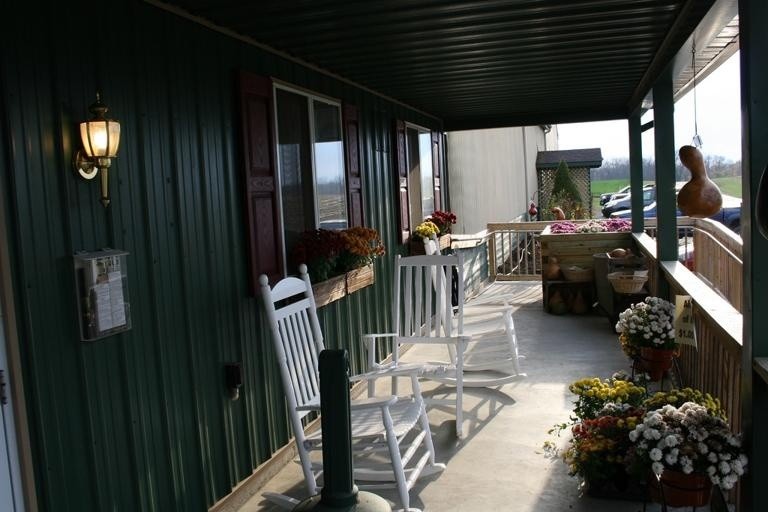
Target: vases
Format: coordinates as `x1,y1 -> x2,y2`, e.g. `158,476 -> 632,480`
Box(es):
649,471 -> 713,508
639,347 -> 673,369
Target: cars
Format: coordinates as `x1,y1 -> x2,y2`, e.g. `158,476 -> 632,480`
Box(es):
599,183 -> 740,237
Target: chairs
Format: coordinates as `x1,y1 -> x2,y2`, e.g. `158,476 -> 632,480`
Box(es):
424,237 -> 527,386
363,249 -> 471,436
260,265 -> 448,512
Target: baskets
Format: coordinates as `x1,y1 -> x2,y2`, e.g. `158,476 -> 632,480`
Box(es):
607,271 -> 648,293
564,265 -> 593,281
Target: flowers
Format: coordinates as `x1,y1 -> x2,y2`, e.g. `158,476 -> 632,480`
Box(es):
298,224 -> 385,282
414,210 -> 457,239
542,370 -> 749,490
615,296 -> 683,360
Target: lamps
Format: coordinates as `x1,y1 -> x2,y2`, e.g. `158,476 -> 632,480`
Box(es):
72,92 -> 121,209
